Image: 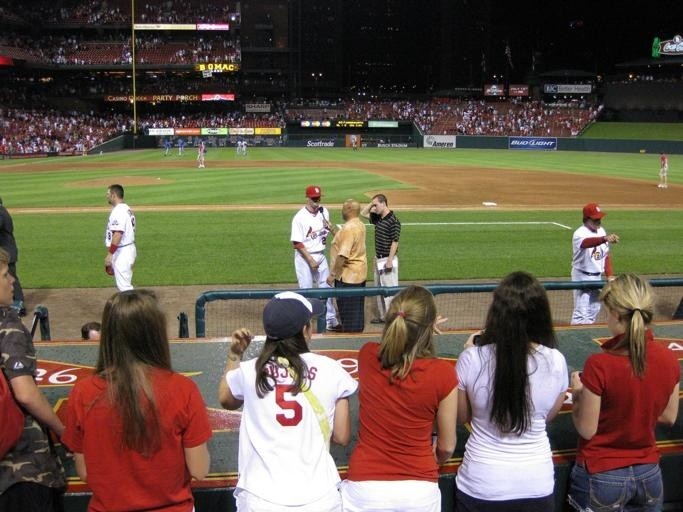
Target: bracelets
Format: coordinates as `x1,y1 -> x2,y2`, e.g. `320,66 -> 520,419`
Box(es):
107,243 -> 119,255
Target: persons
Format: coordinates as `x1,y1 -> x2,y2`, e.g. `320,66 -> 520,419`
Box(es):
558,273 -> 680,511
102,184 -> 139,295
320,198 -> 369,334
289,184 -> 342,335
217,290 -> 360,512
452,271 -> 571,512
0,243 -> 69,512
567,202 -> 621,327
331,282 -> 460,512
361,192 -> 404,326
59,287 -> 213,512
656,151 -> 668,189
0,0 -> 607,168
0,194 -> 27,321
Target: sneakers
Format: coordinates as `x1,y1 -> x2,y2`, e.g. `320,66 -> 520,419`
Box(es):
326,324 -> 342,332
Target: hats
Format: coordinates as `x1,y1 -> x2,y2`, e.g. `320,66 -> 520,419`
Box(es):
263,291 -> 325,339
583,203 -> 607,220
306,185 -> 325,198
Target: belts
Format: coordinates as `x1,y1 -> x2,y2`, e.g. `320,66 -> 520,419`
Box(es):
582,271 -> 601,276
375,253 -> 389,258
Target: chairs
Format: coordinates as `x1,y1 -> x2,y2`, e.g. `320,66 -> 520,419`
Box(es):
281,96 -> 415,129
415,97 -> 605,137
0,1 -> 242,75
0,84 -> 131,156
142,96 -> 284,138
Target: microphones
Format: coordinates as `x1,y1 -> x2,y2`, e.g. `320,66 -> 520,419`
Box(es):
319,206 -> 327,223
